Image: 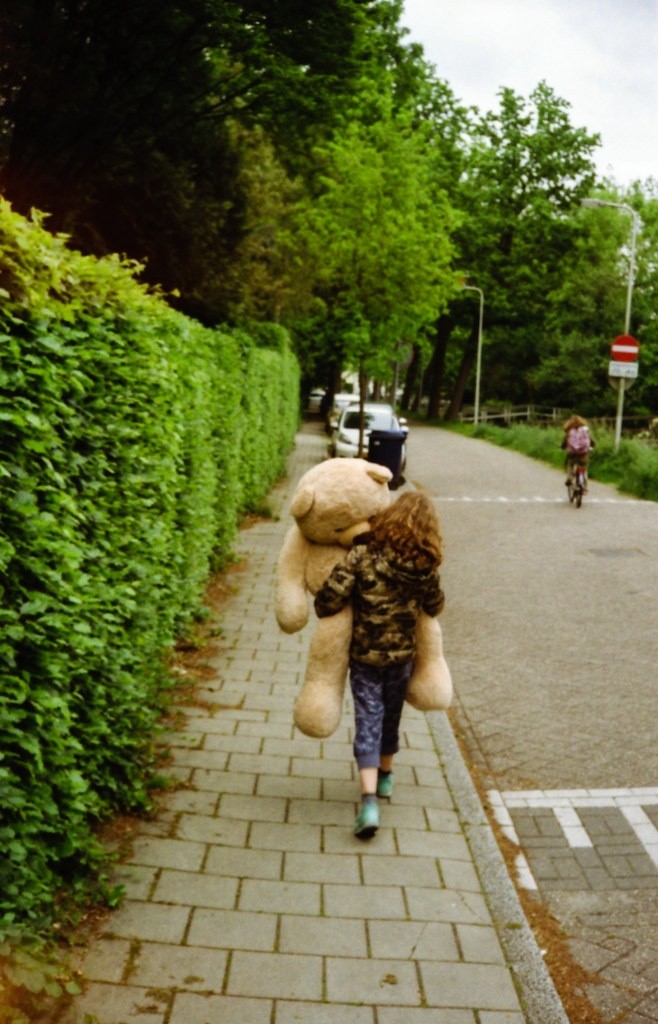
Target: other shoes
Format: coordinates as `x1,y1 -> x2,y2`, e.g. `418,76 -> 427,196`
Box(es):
566,480 -> 571,485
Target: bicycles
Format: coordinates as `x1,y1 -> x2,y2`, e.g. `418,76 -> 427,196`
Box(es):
556,444 -> 595,508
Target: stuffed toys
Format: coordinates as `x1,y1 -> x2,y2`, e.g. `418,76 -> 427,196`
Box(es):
276,457 -> 452,738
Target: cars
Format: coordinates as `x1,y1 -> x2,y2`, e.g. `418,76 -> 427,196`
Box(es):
324,393 -> 362,429
329,400 -> 408,471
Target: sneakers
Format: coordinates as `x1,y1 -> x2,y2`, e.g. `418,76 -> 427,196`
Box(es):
376,770 -> 394,797
355,803 -> 379,836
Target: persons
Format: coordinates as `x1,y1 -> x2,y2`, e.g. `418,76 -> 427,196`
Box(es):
560,415 -> 596,492
314,492 -> 445,837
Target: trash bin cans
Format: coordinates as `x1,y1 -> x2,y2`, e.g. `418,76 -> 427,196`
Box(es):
366,428 -> 408,484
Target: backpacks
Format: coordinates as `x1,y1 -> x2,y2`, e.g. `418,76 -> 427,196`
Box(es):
567,425 -> 590,456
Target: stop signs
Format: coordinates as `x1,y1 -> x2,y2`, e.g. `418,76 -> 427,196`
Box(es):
612,335 -> 640,362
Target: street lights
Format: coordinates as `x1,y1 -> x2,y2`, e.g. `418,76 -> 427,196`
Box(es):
579,197 -> 637,455
